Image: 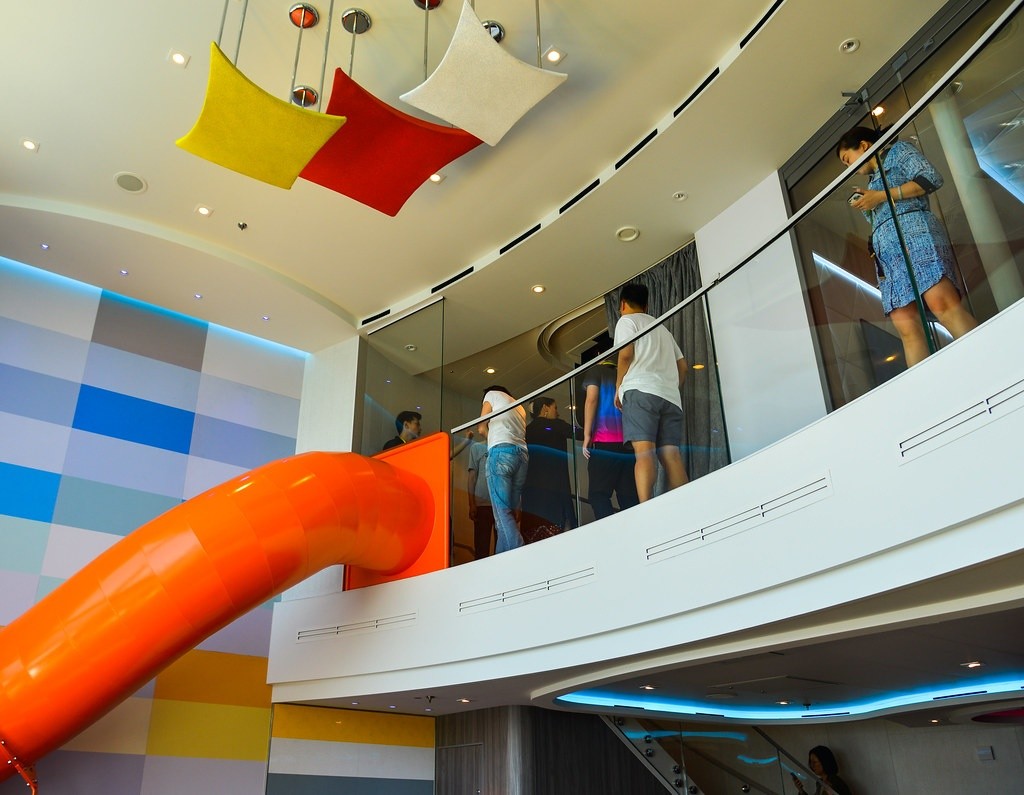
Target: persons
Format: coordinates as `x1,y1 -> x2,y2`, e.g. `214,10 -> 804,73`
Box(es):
835,124 -> 977,368
580,338 -> 641,519
477,385 -> 530,554
614,283 -> 688,504
518,397 -> 585,545
466,437 -> 498,560
791,745 -> 849,795
382,411 -> 474,461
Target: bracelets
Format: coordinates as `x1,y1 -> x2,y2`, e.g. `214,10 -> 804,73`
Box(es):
897,186 -> 903,201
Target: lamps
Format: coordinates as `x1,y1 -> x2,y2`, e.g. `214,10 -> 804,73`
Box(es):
176,0 -> 568,218
